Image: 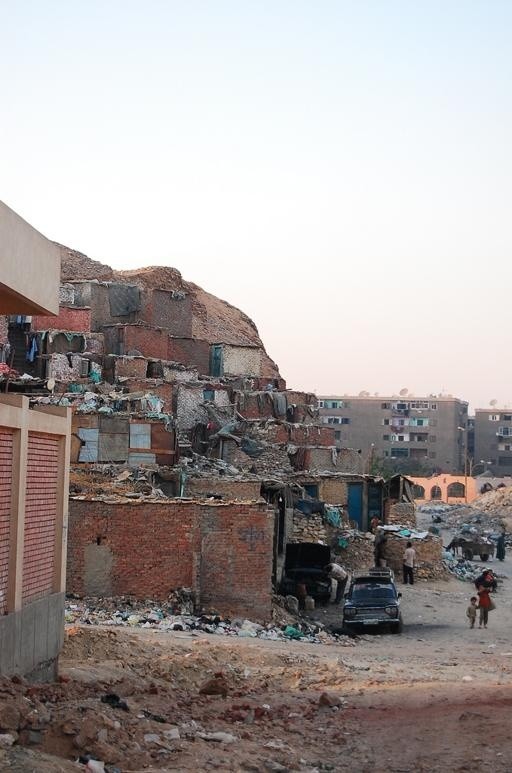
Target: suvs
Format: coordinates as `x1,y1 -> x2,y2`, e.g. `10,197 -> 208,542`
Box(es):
341,566 -> 405,636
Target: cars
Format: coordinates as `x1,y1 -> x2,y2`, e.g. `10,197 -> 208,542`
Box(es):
280,539 -> 334,605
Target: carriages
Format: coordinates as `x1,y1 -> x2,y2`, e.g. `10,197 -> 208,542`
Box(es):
445,536 -> 494,563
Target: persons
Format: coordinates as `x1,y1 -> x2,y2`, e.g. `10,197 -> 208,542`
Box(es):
477,585 -> 490,628
475,569 -> 498,593
324,563 -> 348,604
465,596 -> 479,628
371,514 -> 386,566
402,542 -> 416,585
496,532 -> 506,561
